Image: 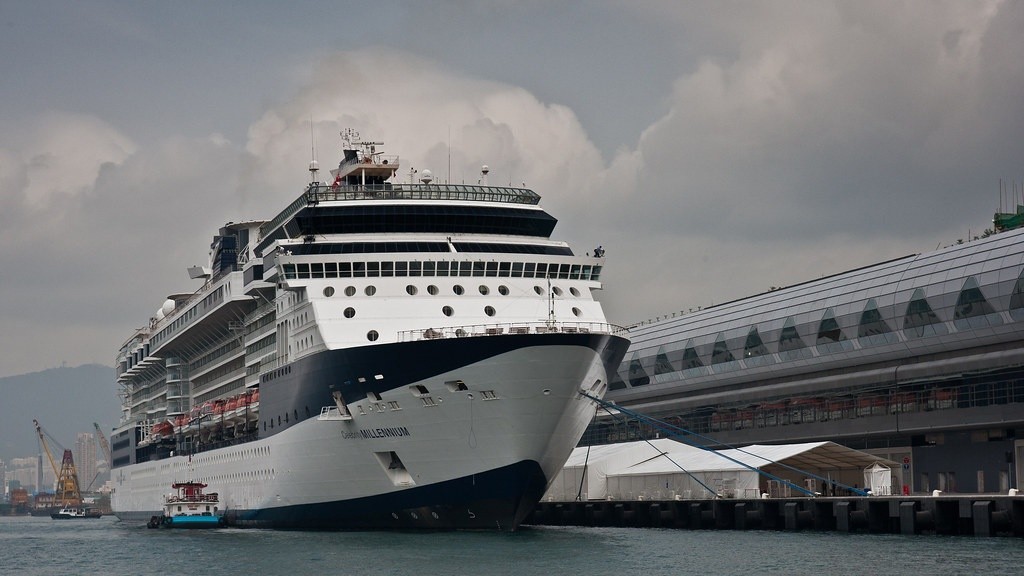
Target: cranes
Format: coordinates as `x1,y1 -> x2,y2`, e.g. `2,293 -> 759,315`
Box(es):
33,418 -> 84,508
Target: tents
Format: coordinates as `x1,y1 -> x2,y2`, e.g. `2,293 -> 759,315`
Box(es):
605,441 -> 903,499
863,461 -> 892,497
540,438 -> 707,501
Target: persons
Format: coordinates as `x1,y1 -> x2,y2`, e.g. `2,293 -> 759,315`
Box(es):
202,437 -> 230,451
821,480 -> 836,496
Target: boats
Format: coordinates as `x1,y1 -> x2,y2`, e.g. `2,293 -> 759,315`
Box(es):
250,389 -> 260,414
147,402 -> 221,442
145,478 -> 230,530
223,397 -> 240,422
235,393 -> 255,418
48,508 -> 86,520
213,400 -> 226,426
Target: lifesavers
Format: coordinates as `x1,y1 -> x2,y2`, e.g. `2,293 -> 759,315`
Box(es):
147,515 -> 173,528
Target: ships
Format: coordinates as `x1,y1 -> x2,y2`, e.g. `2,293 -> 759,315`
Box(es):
104,111 -> 635,538
